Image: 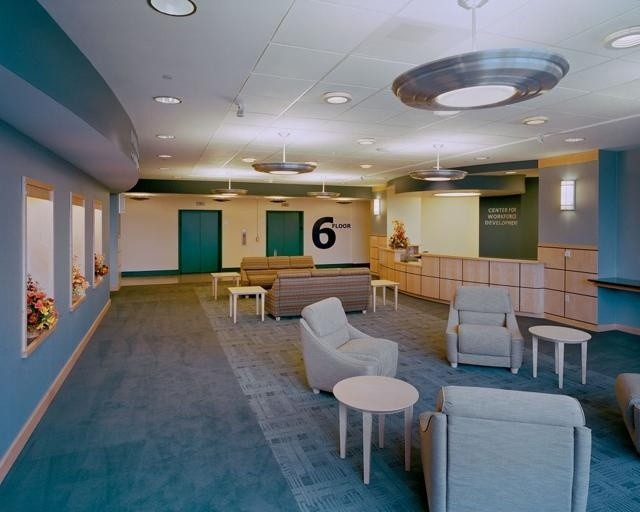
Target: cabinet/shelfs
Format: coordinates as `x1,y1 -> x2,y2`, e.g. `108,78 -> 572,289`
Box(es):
537,244 -> 600,333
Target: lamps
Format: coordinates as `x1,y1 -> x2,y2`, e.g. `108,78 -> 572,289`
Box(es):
392,0 -> 569,111
408,142 -> 468,182
252,131 -> 321,175
212,174 -> 249,198
306,177 -> 341,198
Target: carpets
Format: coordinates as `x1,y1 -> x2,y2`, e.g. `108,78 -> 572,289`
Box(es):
193,285 -> 639,511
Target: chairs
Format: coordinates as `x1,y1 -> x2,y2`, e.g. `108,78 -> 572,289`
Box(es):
445,285 -> 525,375
418,386 -> 592,512
299,296 -> 399,394
615,372 -> 640,457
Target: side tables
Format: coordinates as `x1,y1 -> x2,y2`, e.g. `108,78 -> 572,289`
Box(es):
227,285 -> 267,323
373,279 -> 400,312
211,271 -> 241,301
528,326 -> 593,391
332,374 -> 419,485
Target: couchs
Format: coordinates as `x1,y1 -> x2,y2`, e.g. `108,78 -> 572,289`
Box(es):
241,256 -> 316,298
265,267 -> 372,321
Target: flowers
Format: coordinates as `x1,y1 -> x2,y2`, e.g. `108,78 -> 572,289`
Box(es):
72,255 -> 88,296
24,273 -> 58,332
95,255 -> 109,276
